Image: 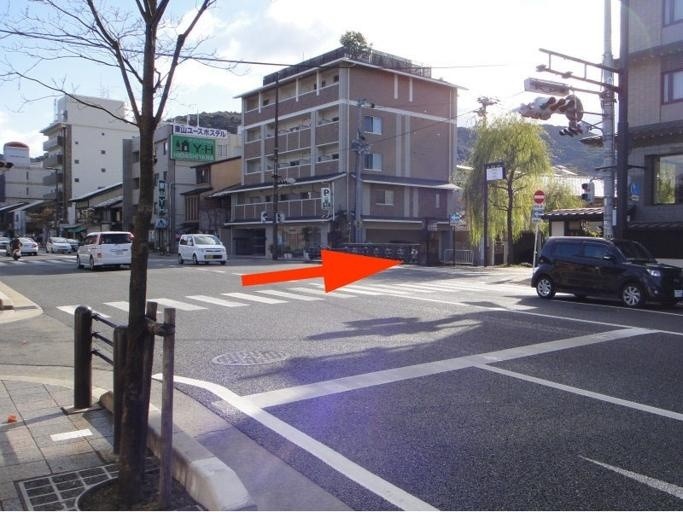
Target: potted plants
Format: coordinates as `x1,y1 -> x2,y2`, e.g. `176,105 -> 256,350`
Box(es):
284,245 -> 294,259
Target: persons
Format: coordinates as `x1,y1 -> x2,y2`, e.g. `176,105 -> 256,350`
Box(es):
8,237 -> 22,250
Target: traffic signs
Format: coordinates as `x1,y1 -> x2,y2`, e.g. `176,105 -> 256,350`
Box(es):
533,207 -> 545,224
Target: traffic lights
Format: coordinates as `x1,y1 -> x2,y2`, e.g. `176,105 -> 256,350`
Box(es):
559,127 -> 581,136
539,96 -> 575,112
581,182 -> 594,202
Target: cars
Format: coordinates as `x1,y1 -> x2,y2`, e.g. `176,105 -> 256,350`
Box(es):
77,231 -> 135,270
179,233 -> 227,265
46,237 -> 79,254
531,236 -> 683,308
1,237 -> 38,255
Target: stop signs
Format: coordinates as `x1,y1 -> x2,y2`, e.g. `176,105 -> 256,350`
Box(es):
534,190 -> 546,205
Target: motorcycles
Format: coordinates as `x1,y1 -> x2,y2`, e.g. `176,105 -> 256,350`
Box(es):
10,246 -> 22,260
